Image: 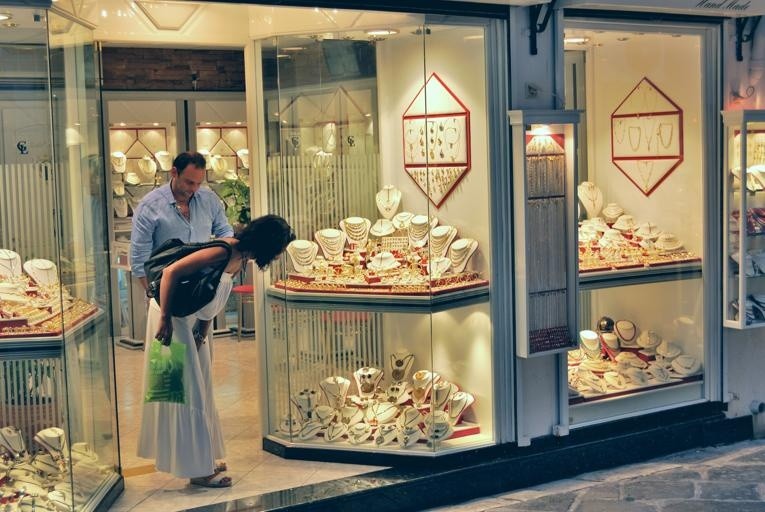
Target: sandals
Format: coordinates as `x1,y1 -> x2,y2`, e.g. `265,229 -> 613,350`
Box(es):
213,463 -> 228,473
191,470 -> 234,489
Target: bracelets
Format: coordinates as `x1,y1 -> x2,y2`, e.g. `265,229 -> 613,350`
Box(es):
276,272 -> 480,292
192,329 -> 205,344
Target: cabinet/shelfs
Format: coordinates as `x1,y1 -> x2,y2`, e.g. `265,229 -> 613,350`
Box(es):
723,110 -> 765,330
196,121 -> 249,342
509,108 -> 579,358
108,125 -> 176,350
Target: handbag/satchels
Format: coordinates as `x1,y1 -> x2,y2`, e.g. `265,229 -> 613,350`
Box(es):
143,237 -> 232,318
145,340 -> 187,404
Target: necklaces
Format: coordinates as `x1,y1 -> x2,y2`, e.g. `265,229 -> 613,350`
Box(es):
637,160 -> 654,191
578,182 -> 680,248
644,118 -> 654,151
659,123 -> 673,148
568,320 -> 697,392
290,184 -> 474,274
31,259 -> 58,289
628,126 -> 641,150
614,118 -> 625,143
279,354 -> 466,447
0,426 -> 109,512
0,249 -> 13,277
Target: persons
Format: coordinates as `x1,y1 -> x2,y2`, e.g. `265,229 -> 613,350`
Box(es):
134,214 -> 299,488
129,151 -> 234,297
